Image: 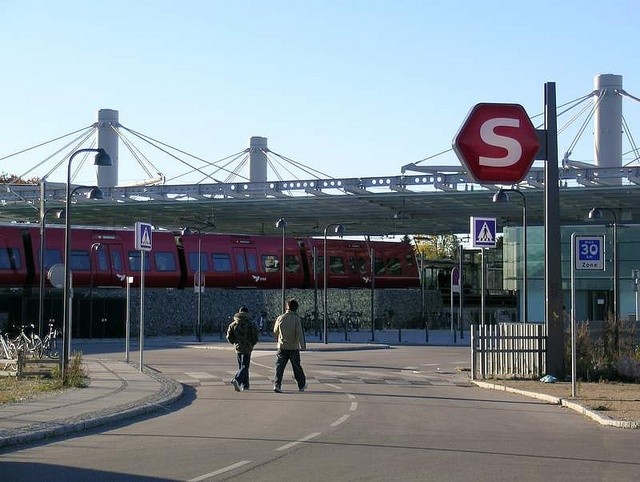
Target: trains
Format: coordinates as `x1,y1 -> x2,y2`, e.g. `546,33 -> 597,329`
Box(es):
0,222 -> 422,290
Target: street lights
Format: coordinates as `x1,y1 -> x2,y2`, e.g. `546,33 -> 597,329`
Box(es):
181,223 -> 216,344
39,206 -> 65,358
276,218 -> 288,314
493,189 -> 527,322
63,149 -> 112,387
323,223 -> 346,343
88,243 -> 105,339
68,186 -> 104,206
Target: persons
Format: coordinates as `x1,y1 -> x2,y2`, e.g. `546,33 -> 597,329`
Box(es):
272,300 -> 306,393
225,307 -> 260,392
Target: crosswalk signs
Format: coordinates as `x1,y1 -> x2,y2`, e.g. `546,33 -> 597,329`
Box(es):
135,222 -> 152,251
471,216 -> 496,248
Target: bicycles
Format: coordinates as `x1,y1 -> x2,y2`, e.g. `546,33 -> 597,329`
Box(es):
0,330 -> 21,371
13,324 -> 43,359
43,318 -> 63,359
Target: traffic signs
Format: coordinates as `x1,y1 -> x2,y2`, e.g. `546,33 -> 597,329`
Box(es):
575,235 -> 603,269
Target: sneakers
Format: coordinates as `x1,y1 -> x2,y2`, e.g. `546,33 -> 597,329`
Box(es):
241,386 -> 250,391
230,377 -> 241,392
298,389 -> 308,394
273,388 -> 283,393
